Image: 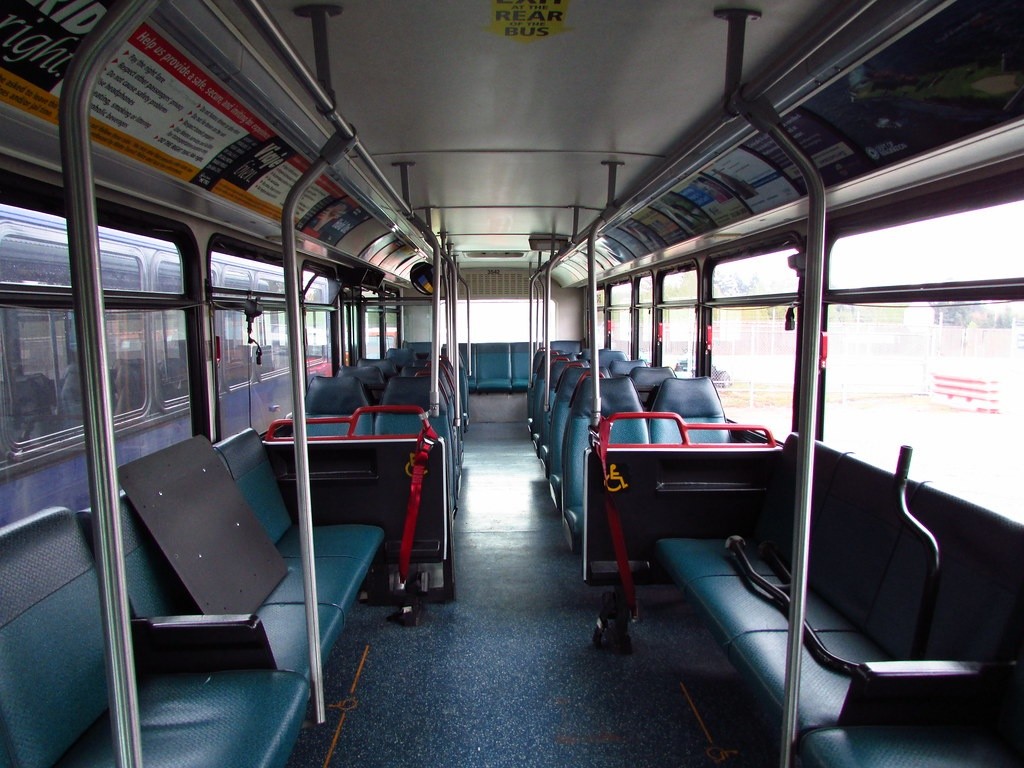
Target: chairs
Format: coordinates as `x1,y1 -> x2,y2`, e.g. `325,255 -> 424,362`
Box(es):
299,347 -> 469,523
9,342 -> 320,441
799,648 -> 1024,768
1,506 -> 310,768
526,348 -> 737,556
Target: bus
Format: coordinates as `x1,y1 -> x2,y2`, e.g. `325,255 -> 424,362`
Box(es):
0,203 -> 349,532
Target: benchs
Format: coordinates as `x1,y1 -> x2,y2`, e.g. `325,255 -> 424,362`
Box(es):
398,339 -> 586,394
643,433 -> 1023,734
75,428 -> 387,688
